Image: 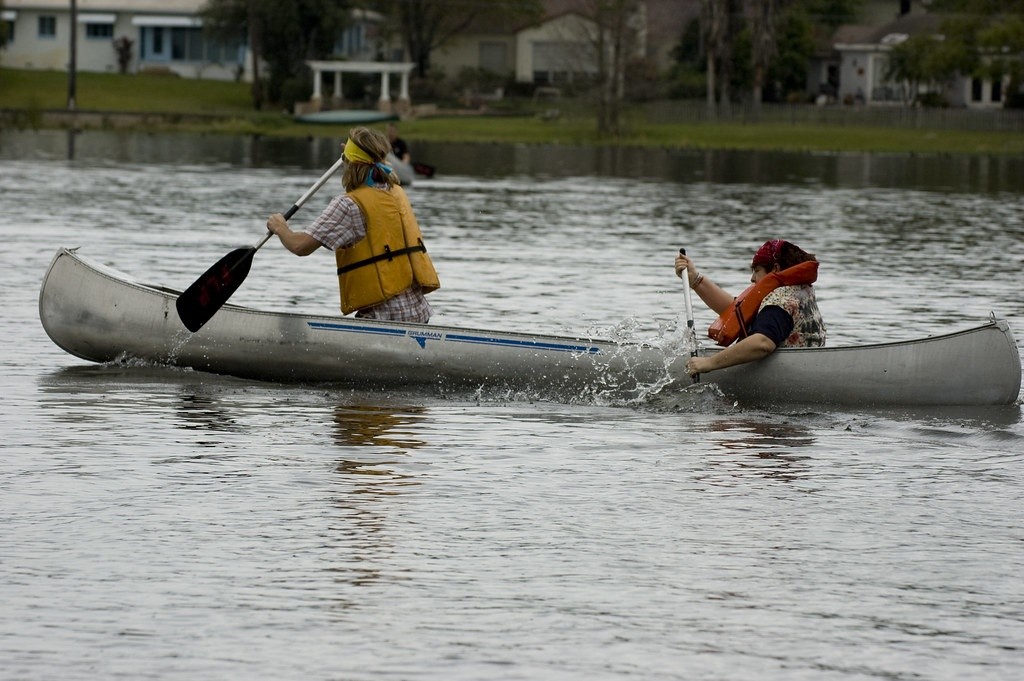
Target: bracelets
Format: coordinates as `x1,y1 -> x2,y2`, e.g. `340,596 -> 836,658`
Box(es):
690,273 -> 704,289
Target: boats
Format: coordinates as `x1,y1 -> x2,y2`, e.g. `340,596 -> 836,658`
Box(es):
39,246 -> 1024,417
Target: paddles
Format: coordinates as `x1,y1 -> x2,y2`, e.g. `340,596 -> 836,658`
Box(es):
174,142 -> 344,333
679,247 -> 701,384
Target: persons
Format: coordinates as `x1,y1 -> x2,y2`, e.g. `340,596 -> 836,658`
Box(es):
268,126 -> 439,325
674,240 -> 827,374
384,124 -> 410,160
